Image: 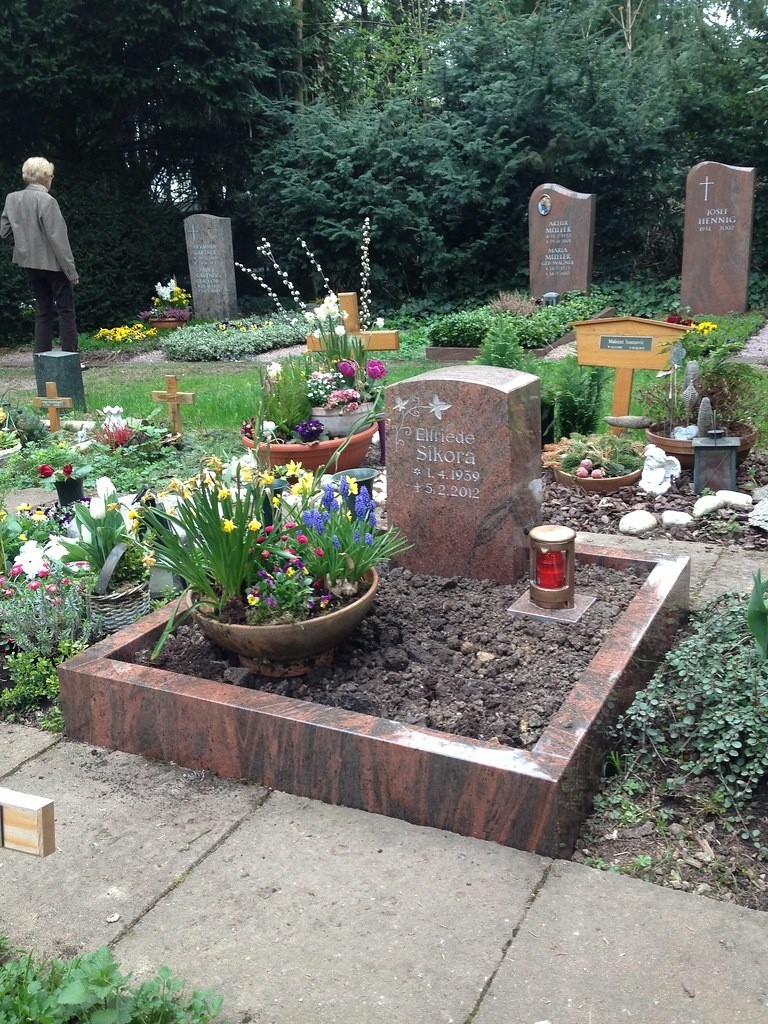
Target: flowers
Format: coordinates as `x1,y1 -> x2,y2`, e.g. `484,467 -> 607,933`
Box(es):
540,432 -> 647,480
241,361 -> 331,448
137,307 -> 192,323
107,452 -> 416,630
304,358 -> 388,417
55,475 -> 151,596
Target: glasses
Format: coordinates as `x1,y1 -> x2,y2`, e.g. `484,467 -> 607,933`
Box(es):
46,174 -> 54,179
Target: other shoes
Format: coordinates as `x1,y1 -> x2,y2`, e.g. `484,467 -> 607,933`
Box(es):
81,365 -> 89,371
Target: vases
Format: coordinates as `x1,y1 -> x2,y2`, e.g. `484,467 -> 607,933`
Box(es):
241,419 -> 379,476
86,579 -> 153,633
186,550 -> 380,677
309,401 -> 378,438
149,317 -> 184,329
553,466 -> 642,494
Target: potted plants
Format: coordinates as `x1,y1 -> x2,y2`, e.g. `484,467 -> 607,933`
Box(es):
633,341 -> 768,473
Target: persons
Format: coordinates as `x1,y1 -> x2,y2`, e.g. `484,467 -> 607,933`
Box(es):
538,197 -> 550,212
0,156 -> 88,371
